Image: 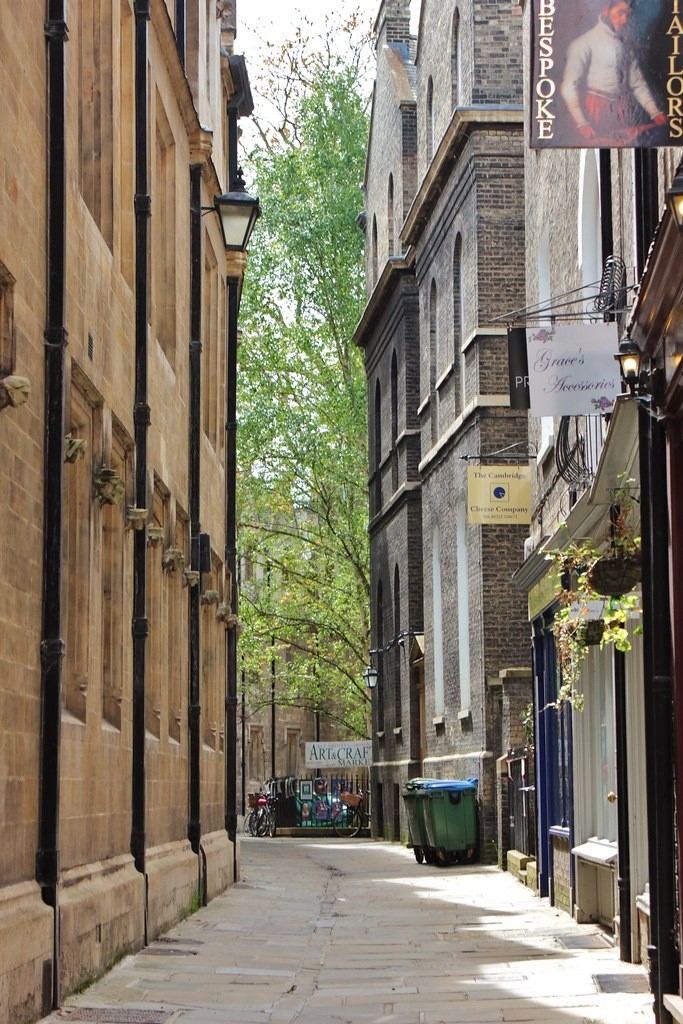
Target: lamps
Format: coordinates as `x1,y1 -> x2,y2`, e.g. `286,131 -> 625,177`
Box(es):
200,166 -> 261,252
664,164 -> 683,236
362,666 -> 380,689
614,326 -> 653,398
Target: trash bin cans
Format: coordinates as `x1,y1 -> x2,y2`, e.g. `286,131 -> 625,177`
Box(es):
401,777 -> 439,864
415,778 -> 479,867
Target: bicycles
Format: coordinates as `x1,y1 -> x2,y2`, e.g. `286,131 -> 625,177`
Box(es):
242,792 -> 279,838
333,783 -> 371,838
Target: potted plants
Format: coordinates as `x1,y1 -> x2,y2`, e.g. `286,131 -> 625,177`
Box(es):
543,471 -> 641,714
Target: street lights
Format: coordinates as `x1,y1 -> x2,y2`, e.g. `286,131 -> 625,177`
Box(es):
212,167 -> 263,891
611,327 -> 680,1024
